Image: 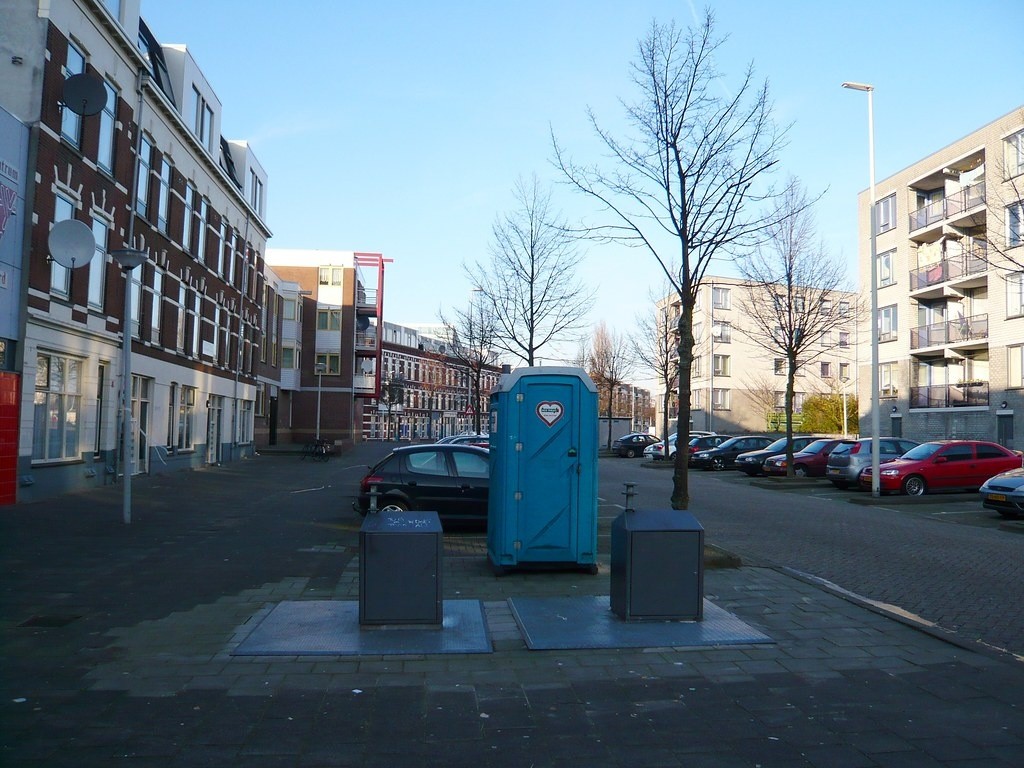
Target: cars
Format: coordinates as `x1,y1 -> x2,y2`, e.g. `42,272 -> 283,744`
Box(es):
826,437 -> 934,489
978,467 -> 1024,519
859,440 -> 1023,496
691,436 -> 776,471
733,437 -> 835,476
762,438 -> 848,478
612,433 -> 662,458
353,432 -> 490,529
643,430 -> 722,463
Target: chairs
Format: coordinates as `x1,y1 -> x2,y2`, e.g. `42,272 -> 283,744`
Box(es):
880,447 -> 887,453
796,444 -> 802,450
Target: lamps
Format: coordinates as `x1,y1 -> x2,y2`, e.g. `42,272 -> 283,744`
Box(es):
892,405 -> 897,412
1002,401 -> 1008,408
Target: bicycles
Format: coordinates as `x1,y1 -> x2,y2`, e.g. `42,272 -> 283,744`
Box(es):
300,433 -> 331,463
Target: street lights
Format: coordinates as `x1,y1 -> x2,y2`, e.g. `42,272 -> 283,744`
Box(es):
315,363 -> 326,444
842,82 -> 880,499
110,249 -> 149,524
468,288 -> 484,435
